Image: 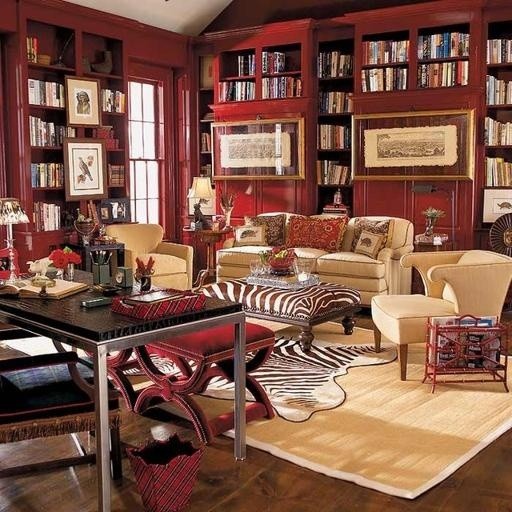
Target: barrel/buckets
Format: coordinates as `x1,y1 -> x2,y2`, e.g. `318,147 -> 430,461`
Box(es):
73,220 -> 97,245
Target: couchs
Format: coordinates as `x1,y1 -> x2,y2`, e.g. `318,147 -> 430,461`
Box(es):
215,211 -> 414,313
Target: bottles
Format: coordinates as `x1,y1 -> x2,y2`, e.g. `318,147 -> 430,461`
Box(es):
333,187 -> 343,204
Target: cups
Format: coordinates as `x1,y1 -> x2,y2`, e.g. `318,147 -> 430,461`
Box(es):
82,232 -> 90,248
248,259 -> 270,276
212,222 -> 220,231
98,224 -> 106,237
140,275 -> 152,293
65,263 -> 75,281
432,233 -> 442,247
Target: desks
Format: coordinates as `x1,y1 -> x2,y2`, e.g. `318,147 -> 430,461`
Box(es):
0,264 -> 247,512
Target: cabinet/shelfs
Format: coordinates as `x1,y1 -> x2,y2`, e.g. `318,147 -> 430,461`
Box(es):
10,1 -> 131,274
310,27 -> 355,214
483,18 -> 512,186
353,14 -> 479,95
195,39 -> 216,183
212,28 -> 316,106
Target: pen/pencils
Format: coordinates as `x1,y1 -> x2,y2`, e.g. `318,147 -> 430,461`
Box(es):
90,250 -> 113,265
136,256 -> 154,274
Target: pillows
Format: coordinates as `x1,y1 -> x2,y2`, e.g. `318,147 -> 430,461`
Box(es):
233,214 -> 395,260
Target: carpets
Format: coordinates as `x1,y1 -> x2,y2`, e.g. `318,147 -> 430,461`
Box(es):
0,320 -> 512,500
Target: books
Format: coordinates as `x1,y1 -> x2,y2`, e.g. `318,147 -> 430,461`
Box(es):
486,39 -> 512,64
317,51 -> 352,184
201,103 -> 215,183
24,35 -> 128,231
417,60 -> 468,88
122,290 -> 187,307
218,50 -> 301,103
486,75 -> 512,105
485,116 -> 512,146
361,41 -> 409,65
428,314 -> 500,370
0,275 -> 89,301
417,32 -> 470,60
484,157 -> 512,186
322,203 -> 351,217
361,68 -> 407,92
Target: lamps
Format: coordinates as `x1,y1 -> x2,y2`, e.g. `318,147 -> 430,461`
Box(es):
0,198 -> 31,280
187,176 -> 214,229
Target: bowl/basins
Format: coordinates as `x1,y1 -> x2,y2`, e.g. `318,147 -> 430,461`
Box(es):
37,55 -> 51,66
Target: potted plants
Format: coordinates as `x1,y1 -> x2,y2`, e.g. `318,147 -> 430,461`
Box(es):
413,208 -> 449,245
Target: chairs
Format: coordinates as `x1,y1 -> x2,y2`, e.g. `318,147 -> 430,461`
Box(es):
0,349 -> 120,482
370,249 -> 512,381
105,223 -> 194,291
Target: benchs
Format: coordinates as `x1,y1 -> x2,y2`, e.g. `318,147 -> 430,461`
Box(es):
84,320 -> 275,443
191,277 -> 362,353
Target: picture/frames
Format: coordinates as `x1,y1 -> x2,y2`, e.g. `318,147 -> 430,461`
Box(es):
64,75 -> 103,129
350,109 -> 477,181
209,118 -> 306,181
480,187 -> 511,227
63,137 -> 109,202
